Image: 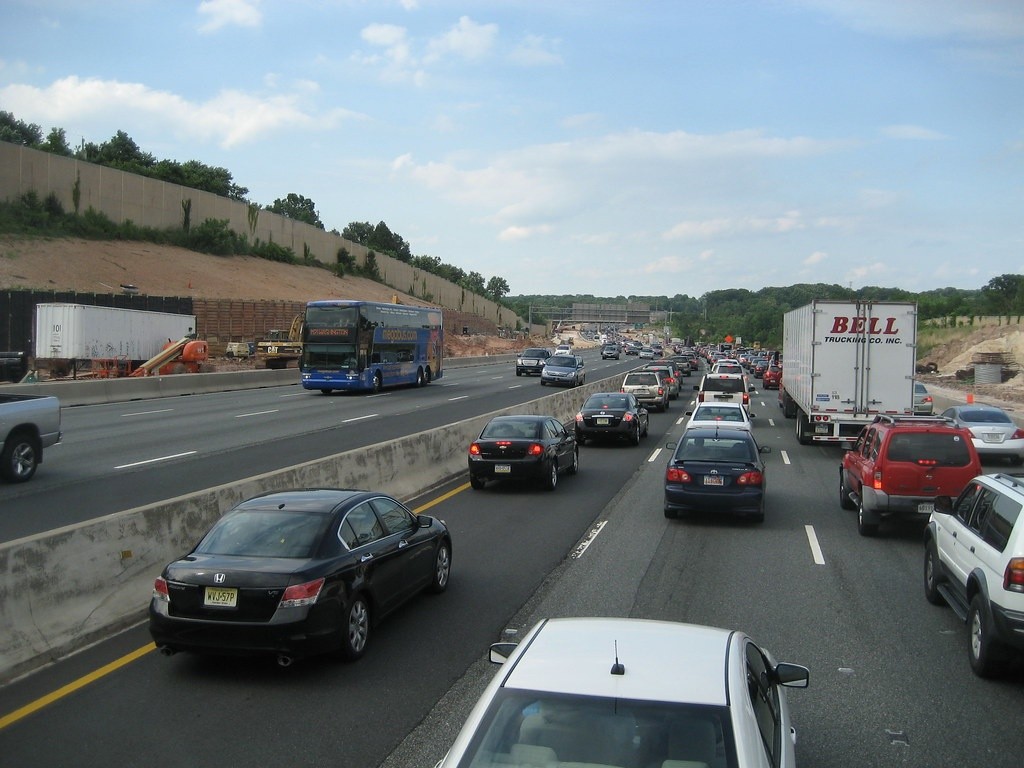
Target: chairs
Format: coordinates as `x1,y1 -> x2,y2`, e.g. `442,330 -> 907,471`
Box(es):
591,398 -> 603,408
611,397 -> 625,408
701,409 -> 712,415
732,443 -> 746,448
694,438 -> 705,446
699,414 -> 712,420
517,697 -> 595,763
731,412 -> 737,415
499,424 -> 513,431
732,382 -> 742,392
631,378 -> 640,384
708,380 -> 723,389
492,429 -> 513,436
724,416 -> 739,421
888,443 -> 913,462
938,439 -> 967,467
525,430 -> 536,438
720,448 -> 747,458
687,446 -> 705,457
529,426 -> 537,431
669,719 -> 716,763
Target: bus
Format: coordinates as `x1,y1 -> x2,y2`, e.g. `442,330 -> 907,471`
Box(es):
298,300 -> 444,394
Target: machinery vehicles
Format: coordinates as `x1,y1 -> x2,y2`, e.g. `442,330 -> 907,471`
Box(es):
92,333 -> 208,380
256,293 -> 397,369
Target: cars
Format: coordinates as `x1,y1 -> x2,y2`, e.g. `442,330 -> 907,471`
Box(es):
575,393 -> 649,446
516,345 -> 586,388
435,617 -> 809,768
667,338 -> 736,376
913,382 -> 1024,466
148,489 -> 453,668
468,415 -> 579,492
664,402 -> 771,525
580,324 -> 665,359
736,347 -> 783,389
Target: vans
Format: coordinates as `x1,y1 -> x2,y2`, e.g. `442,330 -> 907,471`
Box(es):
225,342 -> 255,359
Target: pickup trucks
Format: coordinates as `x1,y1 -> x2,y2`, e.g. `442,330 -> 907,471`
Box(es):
0,394 -> 64,482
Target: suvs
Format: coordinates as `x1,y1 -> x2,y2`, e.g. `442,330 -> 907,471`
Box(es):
923,473 -> 1024,680
692,359 -> 755,413
840,414 -> 983,537
622,358 -> 683,411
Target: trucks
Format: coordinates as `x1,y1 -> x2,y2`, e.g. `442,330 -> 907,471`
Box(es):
779,299 -> 917,445
